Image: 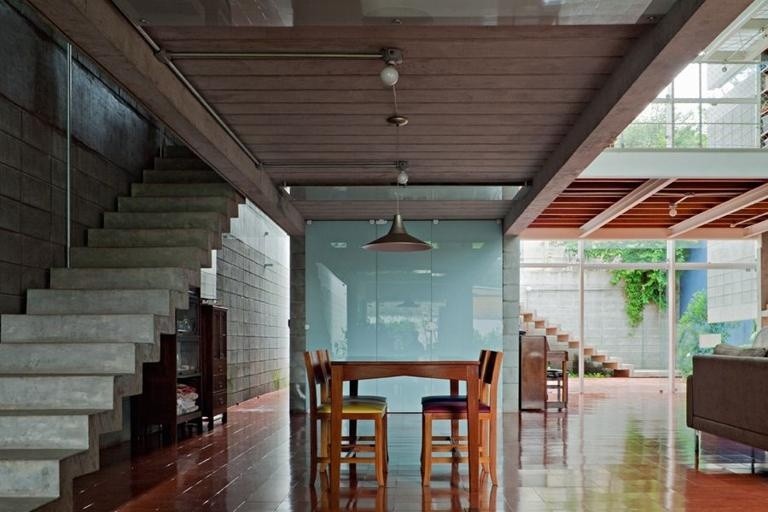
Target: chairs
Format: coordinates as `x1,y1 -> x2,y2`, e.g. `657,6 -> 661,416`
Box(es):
305,348 -> 503,492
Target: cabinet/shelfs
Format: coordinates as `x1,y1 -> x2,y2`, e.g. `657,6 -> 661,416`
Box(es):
755,48 -> 767,150
129,286 -> 228,444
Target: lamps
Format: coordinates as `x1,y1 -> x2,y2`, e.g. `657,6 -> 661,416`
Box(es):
358,66 -> 436,254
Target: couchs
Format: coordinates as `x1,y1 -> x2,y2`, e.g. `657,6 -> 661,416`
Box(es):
684,355 -> 768,452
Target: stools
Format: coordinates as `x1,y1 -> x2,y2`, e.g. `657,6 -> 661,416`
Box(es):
548,369 -> 569,407
